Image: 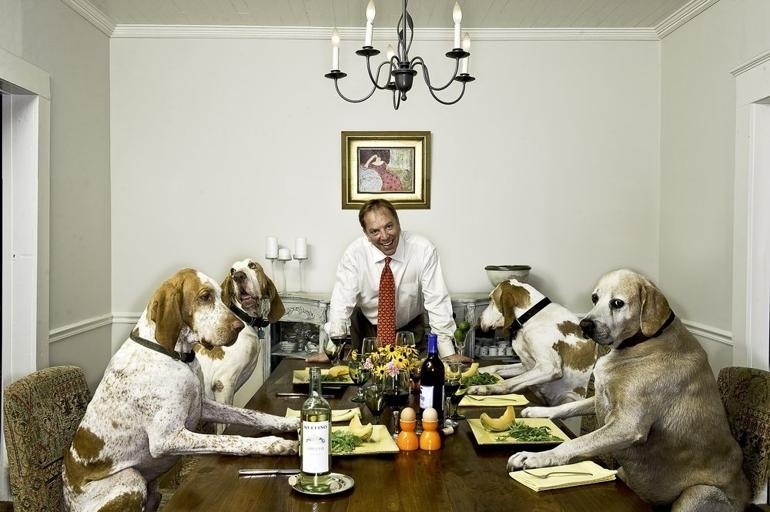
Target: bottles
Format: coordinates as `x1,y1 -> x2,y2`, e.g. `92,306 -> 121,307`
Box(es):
397,420 -> 419,451
418,333 -> 445,428
299,366 -> 333,491
419,419 -> 441,450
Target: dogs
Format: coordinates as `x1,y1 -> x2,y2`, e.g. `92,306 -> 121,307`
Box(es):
193,259 -> 286,434
504,268 -> 751,512
62,268 -> 301,512
466,279 -> 599,438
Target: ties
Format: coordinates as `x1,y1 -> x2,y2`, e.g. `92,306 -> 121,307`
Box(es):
377,258 -> 397,349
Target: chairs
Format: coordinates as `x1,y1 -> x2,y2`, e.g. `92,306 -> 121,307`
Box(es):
713,364 -> 769,509
3,365 -> 91,512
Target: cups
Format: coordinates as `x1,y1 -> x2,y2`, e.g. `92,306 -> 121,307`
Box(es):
475,343 -> 513,356
395,332 -> 415,347
361,337 -> 383,354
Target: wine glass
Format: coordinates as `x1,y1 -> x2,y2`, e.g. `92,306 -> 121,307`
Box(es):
322,339 -> 340,369
444,364 -> 467,421
329,321 -> 347,367
383,370 -> 410,437
349,354 -> 371,403
363,384 -> 388,426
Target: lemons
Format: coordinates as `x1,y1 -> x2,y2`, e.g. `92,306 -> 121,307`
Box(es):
459,321 -> 470,332
454,329 -> 466,340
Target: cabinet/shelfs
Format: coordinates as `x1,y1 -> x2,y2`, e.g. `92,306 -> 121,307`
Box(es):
424,290 -> 523,364
262,293 -> 332,379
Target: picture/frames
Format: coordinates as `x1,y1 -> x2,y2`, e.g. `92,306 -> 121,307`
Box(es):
339,130 -> 434,210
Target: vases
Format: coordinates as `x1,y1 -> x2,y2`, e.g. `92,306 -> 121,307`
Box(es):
485,265 -> 531,286
379,369 -> 411,405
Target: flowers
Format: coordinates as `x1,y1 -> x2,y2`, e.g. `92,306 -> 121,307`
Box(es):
363,343 -> 421,379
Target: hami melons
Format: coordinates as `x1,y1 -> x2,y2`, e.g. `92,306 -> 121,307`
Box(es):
480,405 -> 515,431
349,413 -> 373,440
328,364 -> 350,376
444,363 -> 478,381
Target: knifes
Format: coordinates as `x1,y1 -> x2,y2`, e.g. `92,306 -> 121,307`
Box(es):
276,392 -> 335,399
238,468 -> 300,475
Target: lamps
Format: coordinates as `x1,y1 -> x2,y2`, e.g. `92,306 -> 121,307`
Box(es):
326,0 -> 473,109
264,235 -> 310,297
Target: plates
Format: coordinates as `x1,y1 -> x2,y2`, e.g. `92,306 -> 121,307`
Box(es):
483,371 -> 505,386
296,423 -> 401,456
467,416 -> 573,446
292,367 -> 357,385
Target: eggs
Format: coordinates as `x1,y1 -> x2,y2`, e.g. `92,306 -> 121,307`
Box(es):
422,408 -> 437,421
401,407 -> 415,422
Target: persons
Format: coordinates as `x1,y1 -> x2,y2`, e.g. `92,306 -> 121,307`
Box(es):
304,200 -> 477,365
363,154 -> 404,192
